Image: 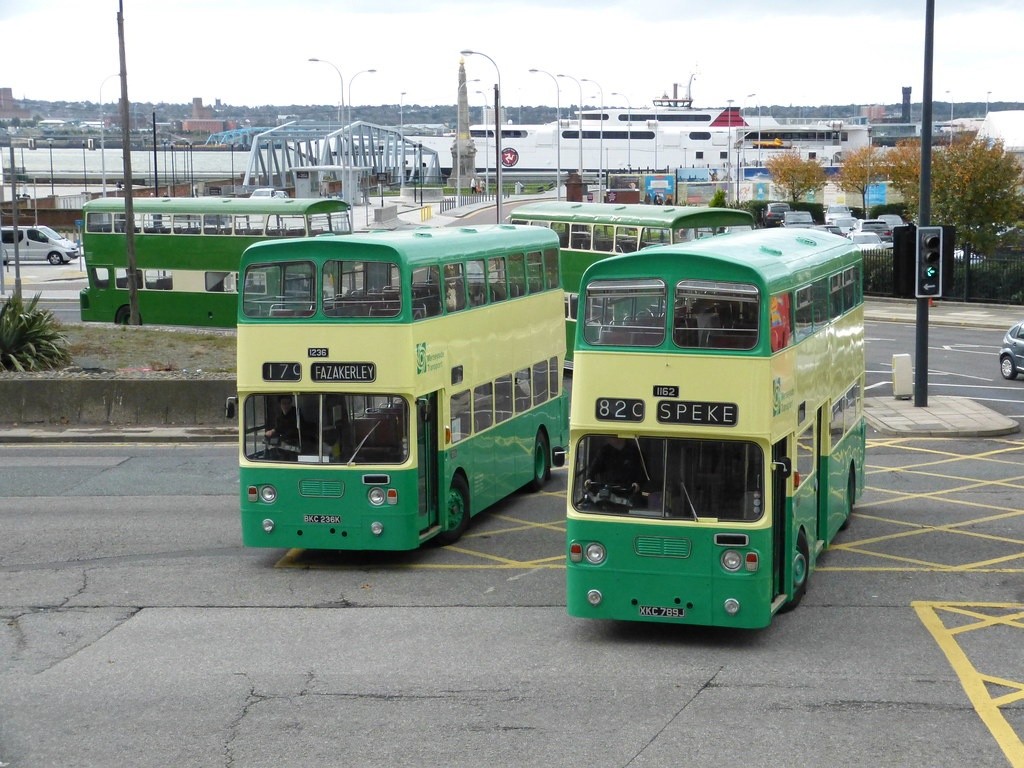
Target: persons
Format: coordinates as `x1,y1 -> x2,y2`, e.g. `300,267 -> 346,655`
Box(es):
644,193 -> 673,205
264,395 -> 304,460
583,438 -> 664,509
471,178 -> 486,194
115,217 -> 126,233
435,279 -> 464,314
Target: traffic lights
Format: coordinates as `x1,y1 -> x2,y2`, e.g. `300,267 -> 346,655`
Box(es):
915,226 -> 942,298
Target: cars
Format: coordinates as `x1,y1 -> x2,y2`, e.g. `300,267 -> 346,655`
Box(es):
809,203 -> 988,268
998,322 -> 1024,381
761,202 -> 791,227
245,187 -> 288,200
779,211 -> 816,230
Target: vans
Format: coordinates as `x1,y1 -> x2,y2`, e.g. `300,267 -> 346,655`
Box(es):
1,225 -> 79,266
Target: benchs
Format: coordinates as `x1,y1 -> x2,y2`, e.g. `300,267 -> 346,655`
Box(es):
600,292 -> 759,349
351,382 -> 519,442
89,222 -> 322,235
558,233 -> 658,254
269,277 -> 551,320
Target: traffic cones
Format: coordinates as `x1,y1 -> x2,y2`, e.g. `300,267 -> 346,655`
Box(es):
928,297 -> 934,308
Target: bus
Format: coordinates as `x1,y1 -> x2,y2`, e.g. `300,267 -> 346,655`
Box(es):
504,198 -> 757,370
78,196 -> 351,333
225,222 -> 571,555
566,226 -> 869,631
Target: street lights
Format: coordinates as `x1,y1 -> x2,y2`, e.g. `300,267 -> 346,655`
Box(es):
555,74 -> 587,187
747,94 -> 761,168
306,57 -> 347,203
610,91 -> 631,177
461,47 -> 506,226
45,137 -> 56,194
476,91 -> 490,199
169,141 -> 177,198
102,73 -> 128,225
161,138 -> 169,185
456,78 -> 482,206
229,139 -> 235,192
944,90 -> 955,149
984,89 -> 993,121
399,91 -> 408,192
349,68 -> 379,212
727,99 -> 735,205
579,78 -> 605,204
527,67 -> 562,203
181,137 -> 195,197
